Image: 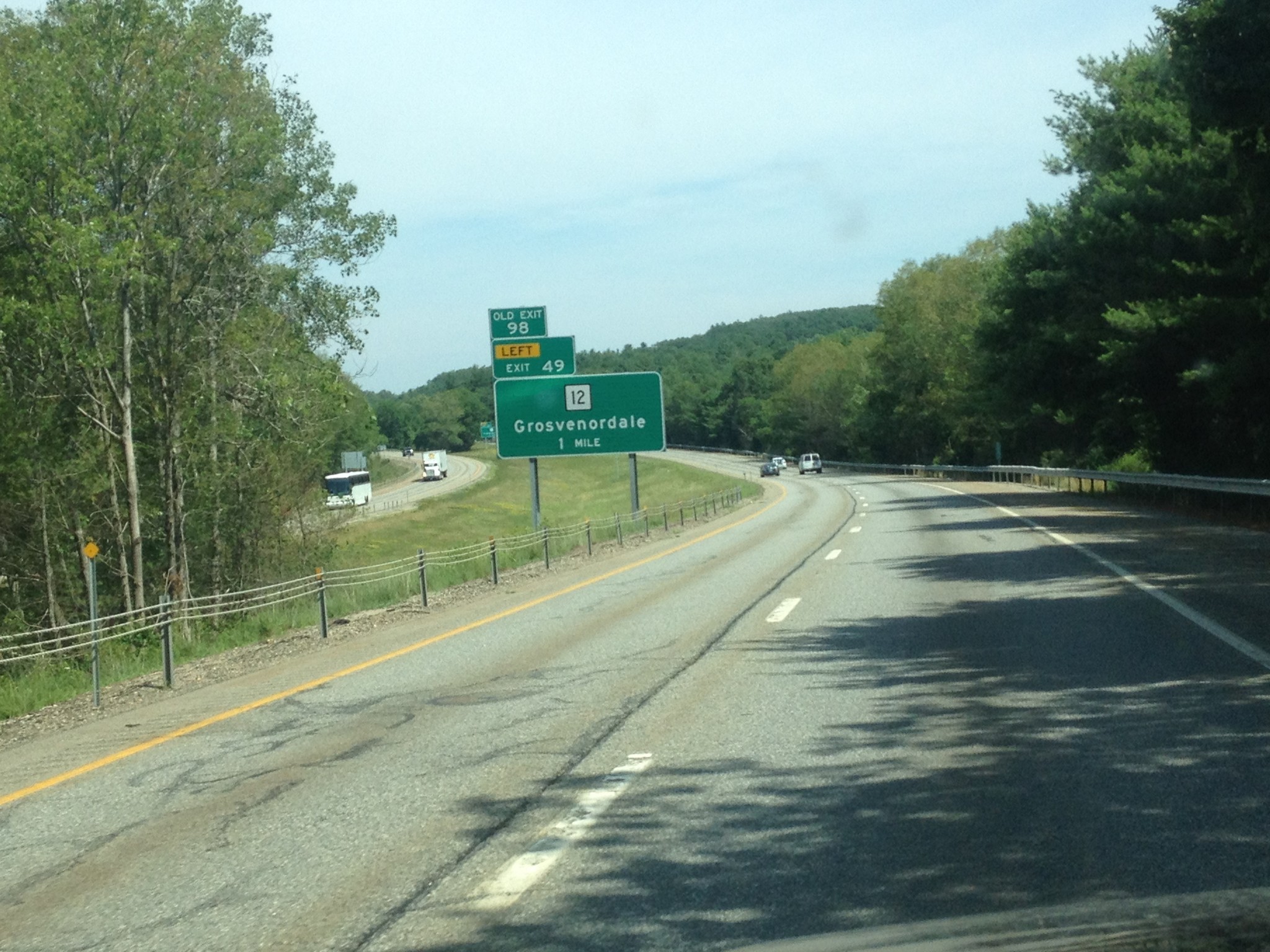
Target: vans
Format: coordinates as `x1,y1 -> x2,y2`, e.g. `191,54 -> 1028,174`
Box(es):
798,453 -> 822,474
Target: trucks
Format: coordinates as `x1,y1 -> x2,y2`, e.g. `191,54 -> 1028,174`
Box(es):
422,450 -> 448,481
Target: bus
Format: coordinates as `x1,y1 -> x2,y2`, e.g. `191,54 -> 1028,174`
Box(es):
325,472 -> 372,509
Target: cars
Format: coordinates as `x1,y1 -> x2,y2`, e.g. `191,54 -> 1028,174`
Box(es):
403,448 -> 415,456
760,463 -> 781,475
773,458 -> 787,469
